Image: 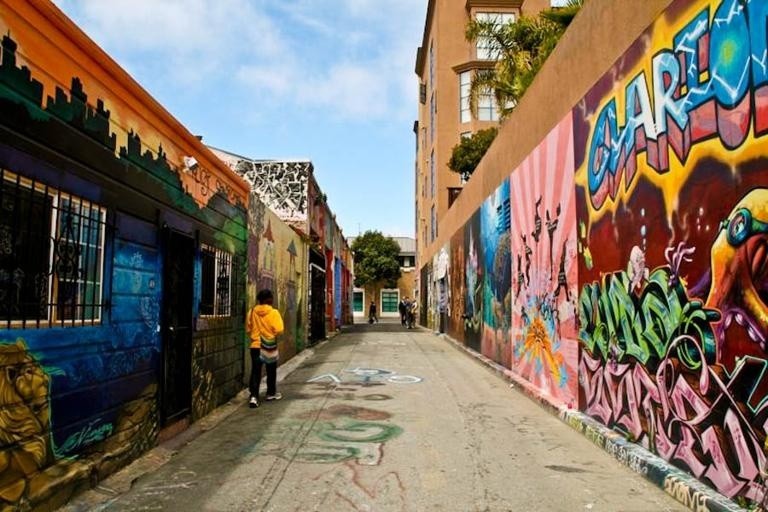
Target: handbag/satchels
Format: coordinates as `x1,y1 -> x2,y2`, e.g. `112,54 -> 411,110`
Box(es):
259,335 -> 279,364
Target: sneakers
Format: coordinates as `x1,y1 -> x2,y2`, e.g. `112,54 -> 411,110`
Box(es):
266,393 -> 282,400
249,397 -> 258,408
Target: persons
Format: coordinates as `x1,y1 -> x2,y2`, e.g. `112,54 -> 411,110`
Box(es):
244,288 -> 285,408
398,295 -> 419,329
368,300 -> 378,323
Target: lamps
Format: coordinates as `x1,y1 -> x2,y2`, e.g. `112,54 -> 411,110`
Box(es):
179,155 -> 199,175
